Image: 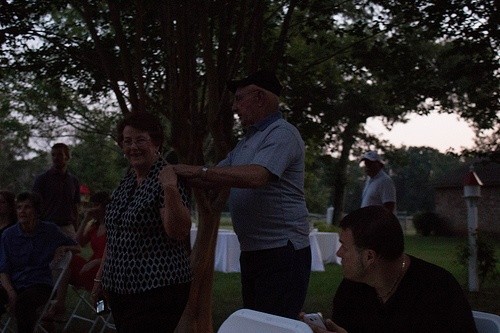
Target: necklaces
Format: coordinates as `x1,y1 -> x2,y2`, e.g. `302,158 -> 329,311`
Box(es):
379,254 -> 406,299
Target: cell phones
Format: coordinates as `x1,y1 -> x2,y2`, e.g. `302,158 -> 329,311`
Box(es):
302,312 -> 328,332
95,297 -> 106,315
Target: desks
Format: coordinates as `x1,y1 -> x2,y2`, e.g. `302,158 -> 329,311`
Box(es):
190,226 -> 343,274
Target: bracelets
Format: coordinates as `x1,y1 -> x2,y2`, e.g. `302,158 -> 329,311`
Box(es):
93,278 -> 101,282
200,165 -> 209,182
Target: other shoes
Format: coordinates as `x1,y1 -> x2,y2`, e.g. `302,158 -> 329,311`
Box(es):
42,305 -> 67,322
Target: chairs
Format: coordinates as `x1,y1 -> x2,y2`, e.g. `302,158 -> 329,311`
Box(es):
2,251 -> 115,333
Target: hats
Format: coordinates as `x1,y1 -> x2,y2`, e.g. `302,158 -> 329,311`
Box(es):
227,70 -> 283,96
359,151 -> 380,162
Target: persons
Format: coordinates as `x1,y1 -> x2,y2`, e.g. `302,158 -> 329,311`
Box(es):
169,72 -> 312,320
358,151 -> 397,209
30,143 -> 80,323
90,110 -> 193,333
0,192 -> 81,333
75,192 -> 110,291
298,206 -> 481,333
0,191 -> 18,315
